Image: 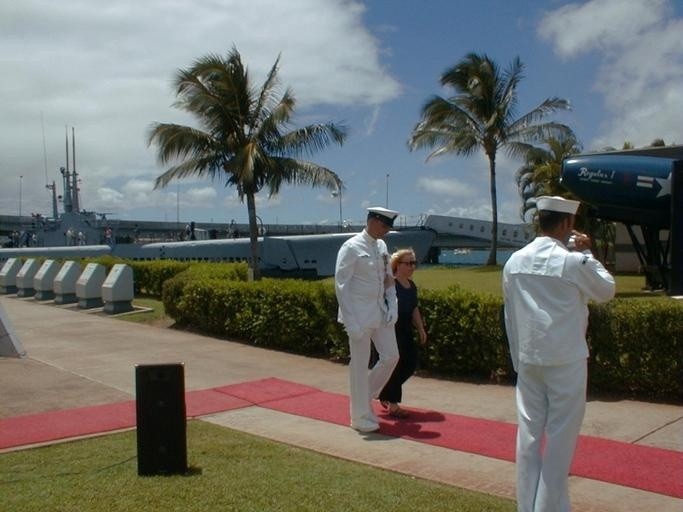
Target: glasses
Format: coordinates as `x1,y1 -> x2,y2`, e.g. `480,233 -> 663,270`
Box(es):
399,261 -> 417,265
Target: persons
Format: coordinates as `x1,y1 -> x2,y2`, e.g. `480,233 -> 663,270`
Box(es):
374,247 -> 428,419
226,219 -> 236,239
102,224 -> 112,245
332,204 -> 401,436
499,194 -> 617,512
132,223 -> 140,243
7,225 -> 87,247
184,222 -> 192,240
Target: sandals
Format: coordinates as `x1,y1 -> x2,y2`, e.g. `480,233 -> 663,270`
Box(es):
379,396 -> 411,418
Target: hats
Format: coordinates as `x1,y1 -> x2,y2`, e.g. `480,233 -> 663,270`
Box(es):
366,208 -> 399,229
535,196 -> 580,216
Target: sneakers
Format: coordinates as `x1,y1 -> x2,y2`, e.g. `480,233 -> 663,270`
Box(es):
350,418 -> 380,432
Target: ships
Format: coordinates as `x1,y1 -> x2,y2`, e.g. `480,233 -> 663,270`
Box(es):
0,125 -> 435,281
